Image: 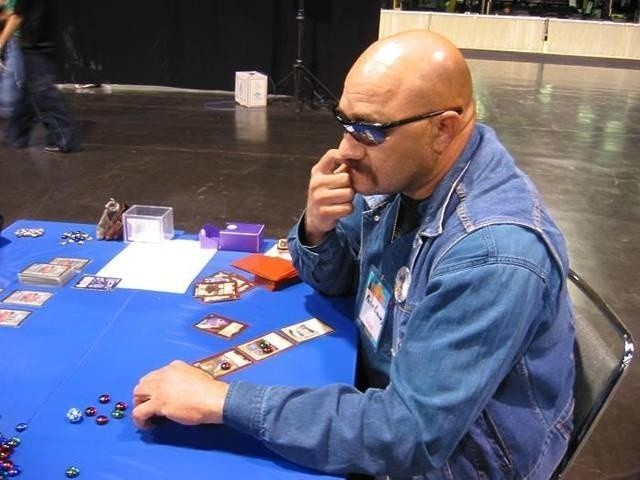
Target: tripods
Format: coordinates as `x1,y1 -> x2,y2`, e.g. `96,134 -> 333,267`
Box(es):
267,0 -> 339,115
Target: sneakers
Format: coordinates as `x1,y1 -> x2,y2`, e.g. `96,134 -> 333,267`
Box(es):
1,128 -> 79,153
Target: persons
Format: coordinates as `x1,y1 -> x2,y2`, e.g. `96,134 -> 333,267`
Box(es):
128,31 -> 580,480
0,0 -> 28,144
0,1 -> 89,153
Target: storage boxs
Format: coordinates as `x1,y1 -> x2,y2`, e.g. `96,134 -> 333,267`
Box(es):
234,70 -> 268,108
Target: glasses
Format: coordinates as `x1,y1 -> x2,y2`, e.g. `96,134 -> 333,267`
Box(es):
333,100 -> 463,145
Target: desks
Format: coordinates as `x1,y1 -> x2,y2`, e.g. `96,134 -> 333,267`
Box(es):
0,219 -> 360,480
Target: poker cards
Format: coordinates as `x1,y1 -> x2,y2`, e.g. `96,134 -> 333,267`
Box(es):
192,270 -> 336,380
0,228 -> 122,327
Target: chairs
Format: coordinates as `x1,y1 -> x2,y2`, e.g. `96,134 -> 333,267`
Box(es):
550,268 -> 636,480
